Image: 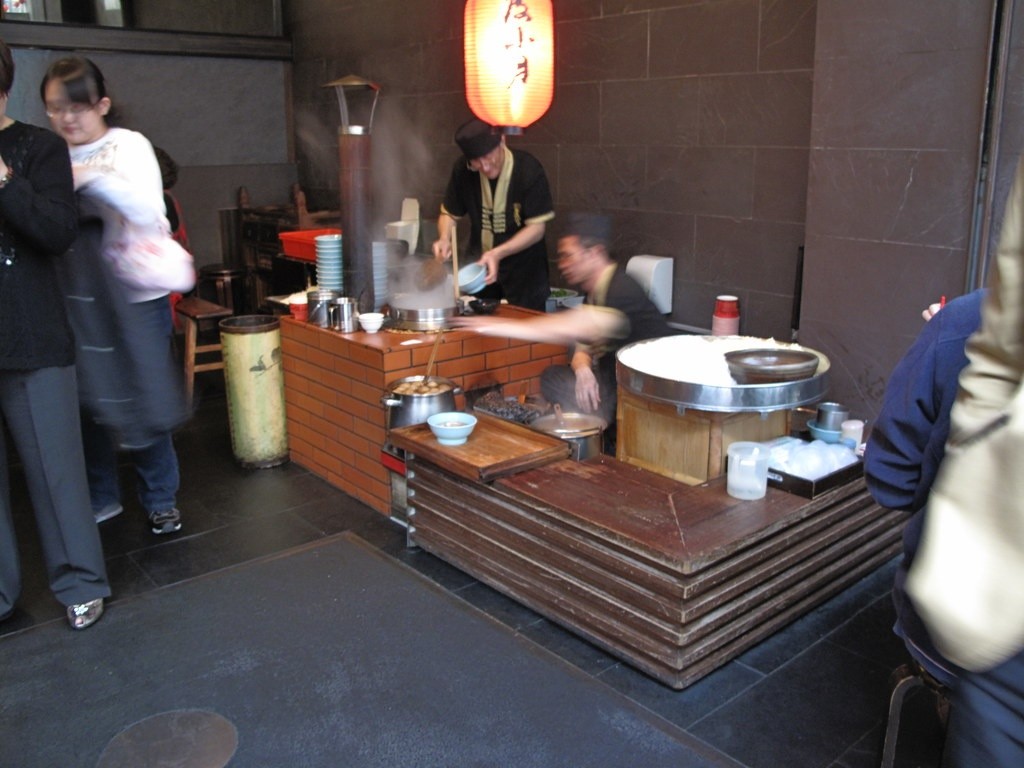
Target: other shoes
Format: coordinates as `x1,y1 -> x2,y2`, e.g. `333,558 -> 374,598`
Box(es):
67,597 -> 104,629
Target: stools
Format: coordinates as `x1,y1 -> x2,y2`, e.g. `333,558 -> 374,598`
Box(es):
196,263 -> 240,309
877,656 -> 954,768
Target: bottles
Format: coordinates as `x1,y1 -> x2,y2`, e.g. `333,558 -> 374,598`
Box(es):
307,291 -> 339,328
842,439 -> 856,452
240,187 -> 249,209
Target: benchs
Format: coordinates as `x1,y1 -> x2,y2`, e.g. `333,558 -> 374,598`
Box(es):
171,296 -> 235,411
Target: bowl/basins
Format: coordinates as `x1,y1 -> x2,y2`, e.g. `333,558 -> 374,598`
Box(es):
427,412 -> 478,447
711,295 -> 740,336
724,349 -> 819,385
315,234 -> 344,296
289,303 -> 308,321
372,242 -> 388,313
807,419 -> 842,444
359,313 -> 385,333
458,262 -> 487,294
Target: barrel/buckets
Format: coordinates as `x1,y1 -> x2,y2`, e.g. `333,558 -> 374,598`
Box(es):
545,287 -> 577,313
200,264 -> 251,316
562,296 -> 584,308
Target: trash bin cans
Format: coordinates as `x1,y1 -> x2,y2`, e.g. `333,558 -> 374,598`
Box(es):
218,314 -> 290,469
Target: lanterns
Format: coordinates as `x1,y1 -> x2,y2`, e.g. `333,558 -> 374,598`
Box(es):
463,0 -> 555,135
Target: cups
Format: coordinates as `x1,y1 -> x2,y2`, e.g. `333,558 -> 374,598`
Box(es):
817,403 -> 850,432
727,442 -> 770,500
841,420 -> 864,454
328,297 -> 362,333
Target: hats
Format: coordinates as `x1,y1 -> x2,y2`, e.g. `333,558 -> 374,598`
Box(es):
455,118 -> 503,161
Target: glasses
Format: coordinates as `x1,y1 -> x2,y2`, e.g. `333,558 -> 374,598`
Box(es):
47,99 -> 101,118
468,147 -> 503,172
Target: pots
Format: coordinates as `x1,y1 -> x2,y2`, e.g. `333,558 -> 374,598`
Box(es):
389,294 -> 464,331
476,396 -> 549,424
526,411 -> 608,460
381,375 -> 463,430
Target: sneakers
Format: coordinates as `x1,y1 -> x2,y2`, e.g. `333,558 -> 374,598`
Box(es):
149,507 -> 182,534
95,503 -> 123,521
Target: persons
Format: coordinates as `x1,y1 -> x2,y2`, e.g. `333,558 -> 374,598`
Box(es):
863,150 -> 1024,768
431,117 -> 554,313
446,212 -> 668,424
0,38 -> 194,629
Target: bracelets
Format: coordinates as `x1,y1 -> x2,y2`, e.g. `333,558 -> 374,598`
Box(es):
0,167 -> 14,189
